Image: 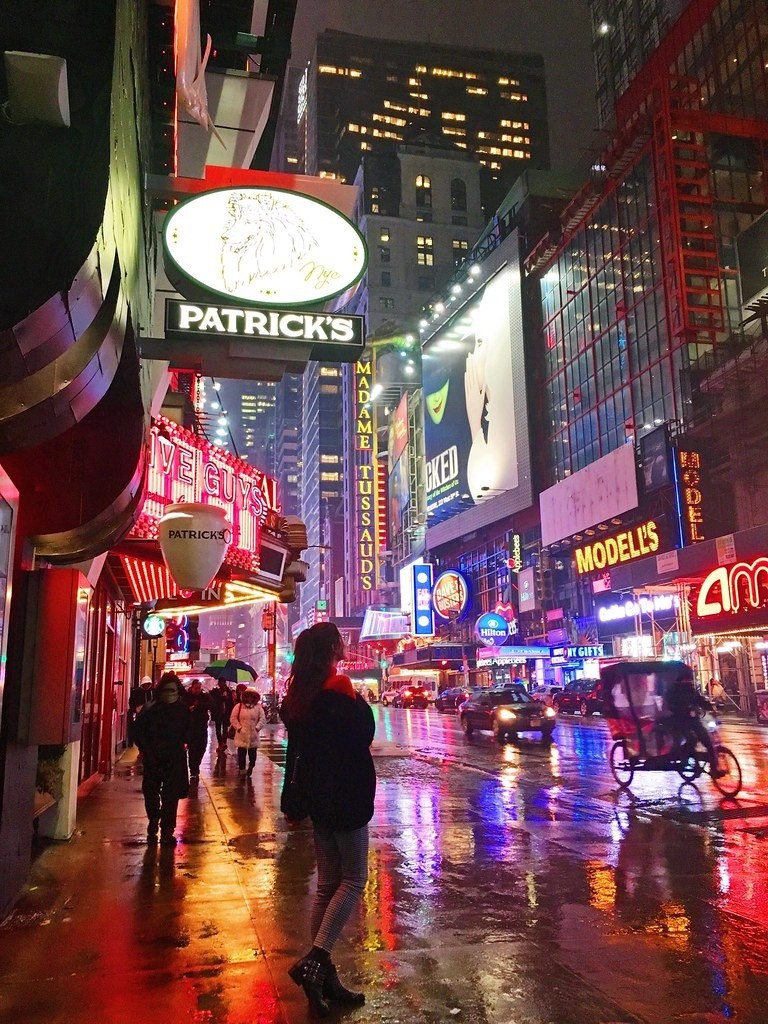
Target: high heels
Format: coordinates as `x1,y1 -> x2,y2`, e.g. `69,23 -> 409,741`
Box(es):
288,949 -> 329,1017
323,963 -> 365,1003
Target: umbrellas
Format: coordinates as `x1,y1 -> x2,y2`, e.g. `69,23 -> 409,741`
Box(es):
204,659 -> 260,689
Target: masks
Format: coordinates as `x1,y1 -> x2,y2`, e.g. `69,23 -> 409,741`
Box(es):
160,690 -> 180,703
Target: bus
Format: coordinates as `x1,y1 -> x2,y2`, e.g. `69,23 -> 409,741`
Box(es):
380,676 -> 438,706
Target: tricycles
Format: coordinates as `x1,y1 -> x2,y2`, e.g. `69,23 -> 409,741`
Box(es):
599,659 -> 744,798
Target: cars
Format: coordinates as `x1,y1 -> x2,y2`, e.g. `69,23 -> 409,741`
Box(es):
550,678 -> 606,717
391,685 -> 428,709
433,687 -> 476,710
458,688 -> 555,742
527,684 -> 566,708
491,683 -> 527,696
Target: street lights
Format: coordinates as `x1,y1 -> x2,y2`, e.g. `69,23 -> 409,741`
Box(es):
267,545 -> 334,726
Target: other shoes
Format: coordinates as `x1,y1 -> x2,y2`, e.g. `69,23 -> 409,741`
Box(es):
247,768 -> 252,776
160,837 -> 176,845
218,738 -> 222,743
190,770 -> 198,779
240,770 -> 247,780
147,835 -> 158,848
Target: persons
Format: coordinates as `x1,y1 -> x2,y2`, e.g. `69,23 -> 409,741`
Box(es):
229,691 -> 266,775
277,622 -> 376,1016
133,673 -> 203,849
134,676 -> 247,779
666,667 -> 727,781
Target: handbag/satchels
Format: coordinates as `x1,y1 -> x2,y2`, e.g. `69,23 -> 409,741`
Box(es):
227,725 -> 236,739
